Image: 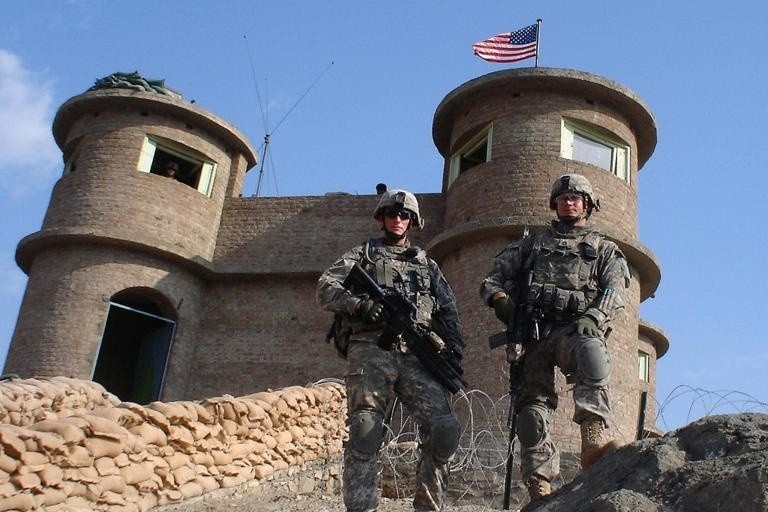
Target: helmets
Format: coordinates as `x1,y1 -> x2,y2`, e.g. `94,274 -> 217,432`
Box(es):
549,173 -> 597,212
371,189 -> 424,231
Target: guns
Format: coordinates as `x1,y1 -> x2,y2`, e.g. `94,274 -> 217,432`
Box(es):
488,267 -> 547,430
341,261 -> 469,393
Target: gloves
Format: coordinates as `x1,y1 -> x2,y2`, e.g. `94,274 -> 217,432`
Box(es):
441,347 -> 464,381
493,295 -> 517,326
358,300 -> 384,323
564,316 -> 604,338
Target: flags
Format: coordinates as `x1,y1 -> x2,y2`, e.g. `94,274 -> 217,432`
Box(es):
472,23 -> 539,64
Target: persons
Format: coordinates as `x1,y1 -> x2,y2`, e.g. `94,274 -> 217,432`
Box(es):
477,173 -> 630,504
164,161 -> 179,179
376,182 -> 387,195
314,188 -> 468,511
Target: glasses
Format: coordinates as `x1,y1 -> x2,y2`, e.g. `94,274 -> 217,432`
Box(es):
384,210 -> 412,221
555,192 -> 584,203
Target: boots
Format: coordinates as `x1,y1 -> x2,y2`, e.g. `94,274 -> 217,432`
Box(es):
580,420 -> 620,471
520,477 -> 551,512
